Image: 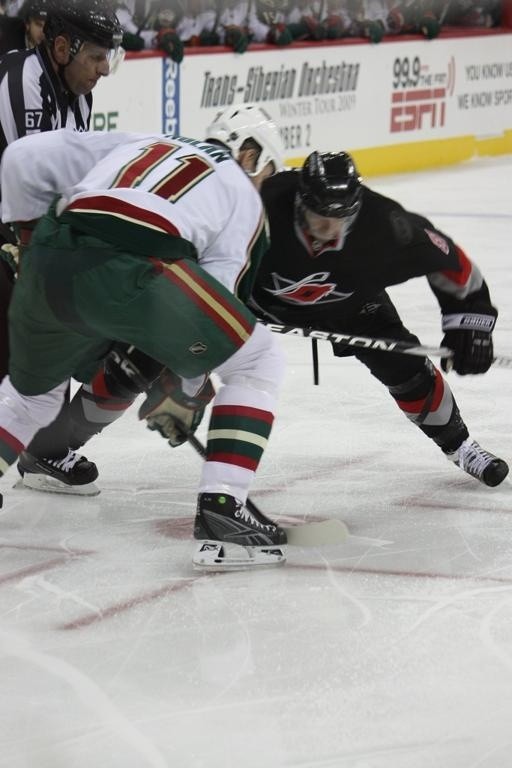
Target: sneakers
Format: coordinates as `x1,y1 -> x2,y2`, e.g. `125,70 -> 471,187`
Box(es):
17,447 -> 99,486
192,493 -> 288,547
448,437 -> 509,488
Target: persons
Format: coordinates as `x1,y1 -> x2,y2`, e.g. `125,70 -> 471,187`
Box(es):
0,0 -> 126,381
0,99 -> 295,549
17,148 -> 511,490
112,1 -> 184,62
444,0 -> 502,28
153,0 -> 440,55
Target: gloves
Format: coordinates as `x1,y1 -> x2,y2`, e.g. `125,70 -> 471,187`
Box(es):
271,15 -> 441,48
157,30 -> 184,65
201,29 -> 221,46
437,303 -> 498,375
137,374 -> 216,449
227,23 -> 249,54
103,347 -> 161,399
119,30 -> 145,52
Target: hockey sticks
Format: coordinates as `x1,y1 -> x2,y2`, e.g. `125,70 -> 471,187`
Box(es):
109,343 -> 348,548
257,318 -> 511,368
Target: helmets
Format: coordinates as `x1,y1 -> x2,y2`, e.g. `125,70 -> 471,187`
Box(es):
18,0 -> 46,28
205,103 -> 285,180
296,151 -> 361,217
44,0 -> 126,49
